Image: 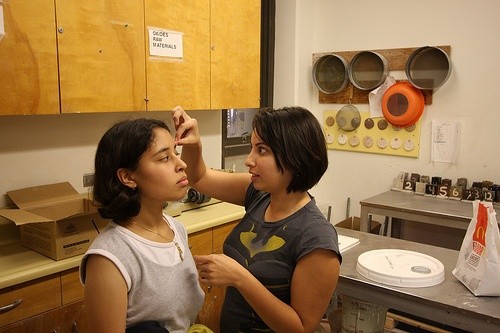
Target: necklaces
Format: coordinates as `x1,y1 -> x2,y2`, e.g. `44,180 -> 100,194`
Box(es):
130,216 -> 183,262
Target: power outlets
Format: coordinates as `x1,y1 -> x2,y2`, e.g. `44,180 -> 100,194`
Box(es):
83,174 -> 94,187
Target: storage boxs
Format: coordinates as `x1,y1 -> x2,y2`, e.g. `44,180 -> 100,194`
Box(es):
336,216 -> 381,235
0,182 -> 113,261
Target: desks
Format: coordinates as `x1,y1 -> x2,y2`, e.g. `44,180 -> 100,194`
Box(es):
334,226 -> 500,333
360,190 -> 500,233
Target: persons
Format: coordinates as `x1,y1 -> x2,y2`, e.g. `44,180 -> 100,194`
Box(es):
79,119 -> 206,333
171,105 -> 342,333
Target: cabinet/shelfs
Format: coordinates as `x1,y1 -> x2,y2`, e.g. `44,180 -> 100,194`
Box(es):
0,266 -> 90,333
0,0 -> 148,115
144,0 -> 262,111
189,219 -> 240,333
